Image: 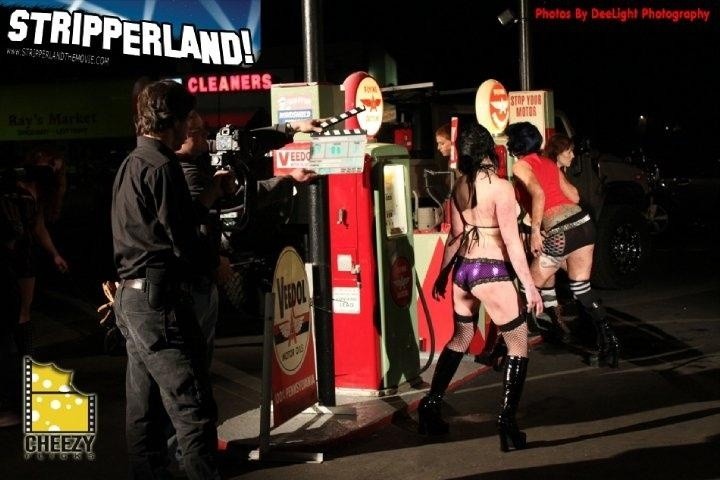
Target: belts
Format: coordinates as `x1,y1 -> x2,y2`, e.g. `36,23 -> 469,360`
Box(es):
119,279 -> 143,290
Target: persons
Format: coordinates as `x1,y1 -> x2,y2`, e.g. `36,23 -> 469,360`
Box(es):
418,122 -> 545,452
1,151 -> 70,357
419,119 -> 620,454
109,77 -> 232,480
175,109 -> 333,479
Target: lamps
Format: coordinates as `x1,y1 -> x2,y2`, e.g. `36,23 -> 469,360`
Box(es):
499,10 -> 521,26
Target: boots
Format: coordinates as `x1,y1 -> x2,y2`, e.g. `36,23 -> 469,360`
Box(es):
418,346 -> 464,427
497,355 -> 526,451
589,298 -> 621,369
